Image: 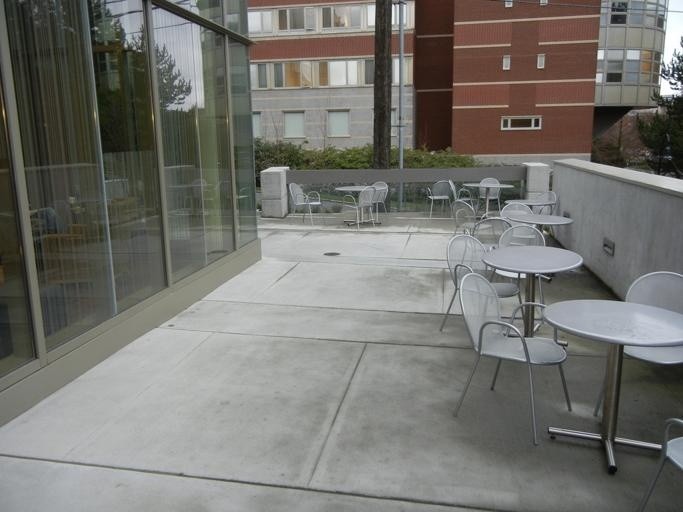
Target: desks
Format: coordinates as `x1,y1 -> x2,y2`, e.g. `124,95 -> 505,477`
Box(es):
333,185 -> 386,226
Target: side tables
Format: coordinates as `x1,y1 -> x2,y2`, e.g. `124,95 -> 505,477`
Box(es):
541,299 -> 682,477
504,198 -> 556,213
482,245 -> 584,347
507,215 -> 574,283
461,182 -> 515,218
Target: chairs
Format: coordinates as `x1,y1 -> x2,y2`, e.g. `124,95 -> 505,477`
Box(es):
340,180 -> 387,231
287,182 -> 327,226
425,179 -> 545,278
439,234 -> 528,331
453,272 -> 573,447
638,418 -> 683,510
591,270 -> 682,419
